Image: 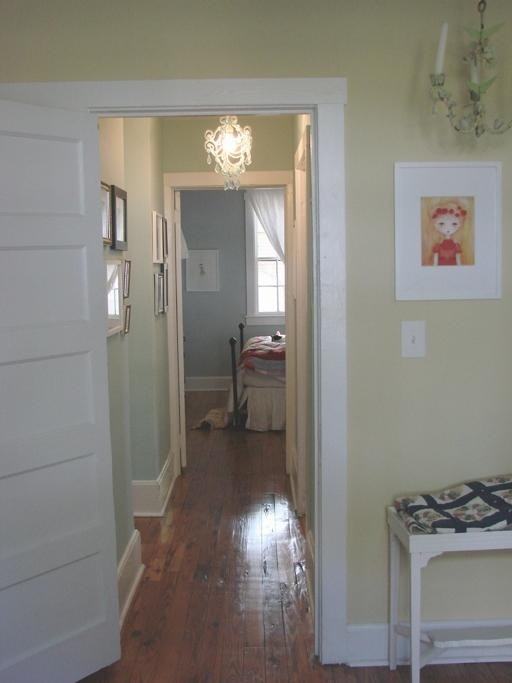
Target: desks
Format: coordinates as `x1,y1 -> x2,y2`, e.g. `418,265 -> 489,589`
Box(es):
388,504 -> 512,681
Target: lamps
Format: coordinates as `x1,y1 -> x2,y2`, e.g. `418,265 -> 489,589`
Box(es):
426,2 -> 511,138
203,115 -> 253,191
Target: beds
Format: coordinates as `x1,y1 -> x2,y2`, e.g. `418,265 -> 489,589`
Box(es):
229,324 -> 286,432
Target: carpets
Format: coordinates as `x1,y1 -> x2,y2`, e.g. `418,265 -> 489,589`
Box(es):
191,408 -> 230,431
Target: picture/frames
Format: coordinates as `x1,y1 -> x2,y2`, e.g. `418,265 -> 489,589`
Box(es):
99,180 -> 168,337
392,162 -> 502,302
186,249 -> 219,291
